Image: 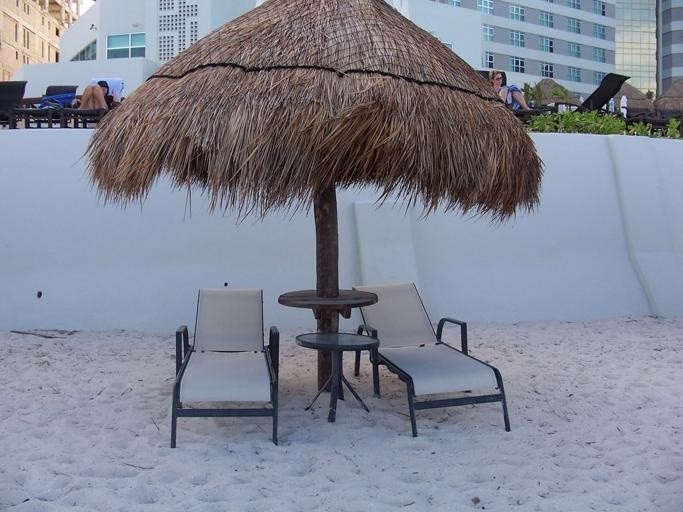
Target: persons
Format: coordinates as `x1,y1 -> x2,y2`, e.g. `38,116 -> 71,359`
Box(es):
71,80 -> 116,113
491,70 -> 533,110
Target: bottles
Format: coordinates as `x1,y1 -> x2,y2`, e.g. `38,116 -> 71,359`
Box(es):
609,98 -> 615,113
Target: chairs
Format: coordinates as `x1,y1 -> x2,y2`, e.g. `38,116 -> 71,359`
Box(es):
483,71 -> 682,138
350,281 -> 511,438
0,78 -> 123,127
170,287 -> 280,447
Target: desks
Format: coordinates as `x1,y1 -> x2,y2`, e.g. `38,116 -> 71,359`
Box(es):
277,289 -> 378,391
294,331 -> 380,422
